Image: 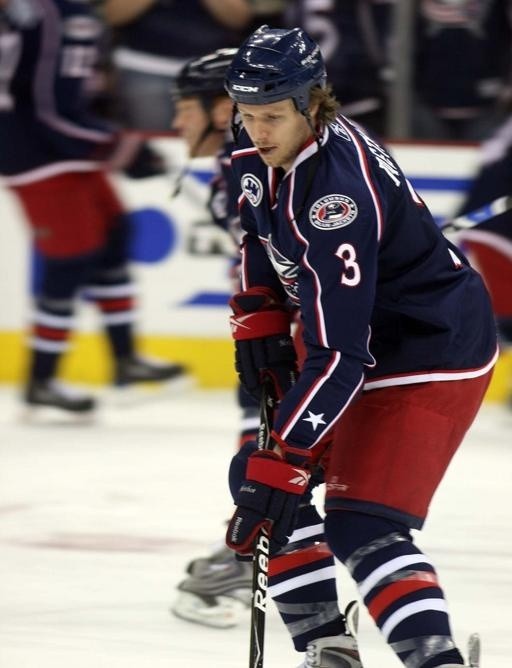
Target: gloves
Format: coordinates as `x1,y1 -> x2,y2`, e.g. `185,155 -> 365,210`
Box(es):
226,294 -> 296,399
225,442 -> 311,563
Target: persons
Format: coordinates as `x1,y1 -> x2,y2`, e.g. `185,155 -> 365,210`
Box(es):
448,118 -> 512,343
212,22 -> 503,666
163,45 -> 318,629
203,0 -> 512,143
0,0 -> 192,411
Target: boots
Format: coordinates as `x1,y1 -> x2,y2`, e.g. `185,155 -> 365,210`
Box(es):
432,629 -> 482,667
292,601 -> 364,668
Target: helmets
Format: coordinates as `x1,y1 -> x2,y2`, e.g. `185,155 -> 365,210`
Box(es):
174,50 -> 242,103
220,23 -> 328,106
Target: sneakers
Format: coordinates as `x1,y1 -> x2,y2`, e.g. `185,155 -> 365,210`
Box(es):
24,375 -> 97,413
108,359 -> 187,389
